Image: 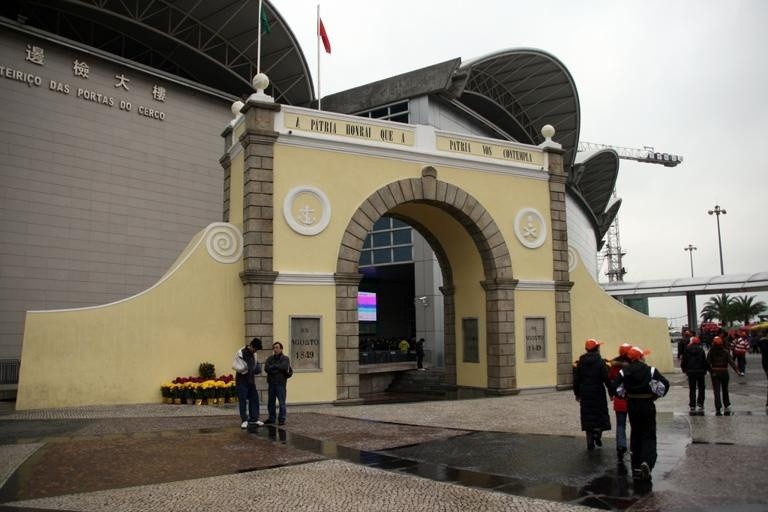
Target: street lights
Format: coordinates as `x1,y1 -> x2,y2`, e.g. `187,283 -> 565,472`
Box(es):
685,243 -> 696,277
708,205 -> 730,274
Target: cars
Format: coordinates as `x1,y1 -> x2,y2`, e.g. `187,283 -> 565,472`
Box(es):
668,330 -> 684,343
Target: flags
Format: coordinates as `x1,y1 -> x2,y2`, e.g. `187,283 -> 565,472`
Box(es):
319,18 -> 332,53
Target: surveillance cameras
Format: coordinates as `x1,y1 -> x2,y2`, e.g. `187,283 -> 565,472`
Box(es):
418,296 -> 427,301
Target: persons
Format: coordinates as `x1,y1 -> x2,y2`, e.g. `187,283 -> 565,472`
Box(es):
611,346 -> 671,487
677,328 -> 768,377
679,336 -> 707,415
359,335 -> 416,363
230,338 -> 265,431
414,337 -> 427,371
262,340 -> 293,426
572,338 -> 613,450
607,343 -> 635,460
706,334 -> 742,416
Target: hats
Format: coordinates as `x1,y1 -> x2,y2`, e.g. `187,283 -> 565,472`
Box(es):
712,336 -> 723,346
687,336 -> 701,347
585,339 -> 650,361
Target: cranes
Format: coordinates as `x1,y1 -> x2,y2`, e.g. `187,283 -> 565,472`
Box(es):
576,137 -> 684,284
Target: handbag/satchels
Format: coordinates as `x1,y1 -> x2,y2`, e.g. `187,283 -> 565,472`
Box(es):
649,366 -> 665,397
284,367 -> 293,378
616,369 -> 628,399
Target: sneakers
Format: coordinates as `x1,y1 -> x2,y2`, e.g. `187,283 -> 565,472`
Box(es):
588,436 -> 602,449
690,402 -> 704,410
241,419 -> 284,428
633,462 -> 651,481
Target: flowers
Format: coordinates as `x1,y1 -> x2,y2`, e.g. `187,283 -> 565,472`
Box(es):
158,373 -> 238,400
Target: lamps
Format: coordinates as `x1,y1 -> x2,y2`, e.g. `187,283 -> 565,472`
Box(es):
536,122 -> 562,149
230,101 -> 244,125
243,71 -> 276,102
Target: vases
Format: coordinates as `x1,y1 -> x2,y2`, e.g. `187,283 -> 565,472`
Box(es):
162,397 -> 239,408
